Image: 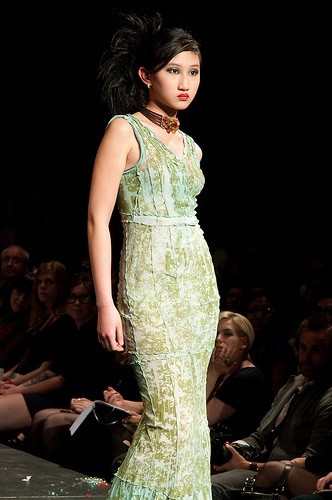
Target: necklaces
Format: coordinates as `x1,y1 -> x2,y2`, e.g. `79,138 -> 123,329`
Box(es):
139,105 -> 180,135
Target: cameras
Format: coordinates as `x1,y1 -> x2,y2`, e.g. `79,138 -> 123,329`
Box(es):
210,438 -> 265,467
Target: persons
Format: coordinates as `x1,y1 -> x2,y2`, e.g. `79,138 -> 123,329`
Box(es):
86,10 -> 220,499
0,243 -> 332,500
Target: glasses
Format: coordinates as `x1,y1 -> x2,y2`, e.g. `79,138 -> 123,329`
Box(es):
65,294 -> 93,304
249,304 -> 275,314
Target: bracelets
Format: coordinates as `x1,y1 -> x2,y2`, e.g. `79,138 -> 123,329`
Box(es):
249,461 -> 258,471
